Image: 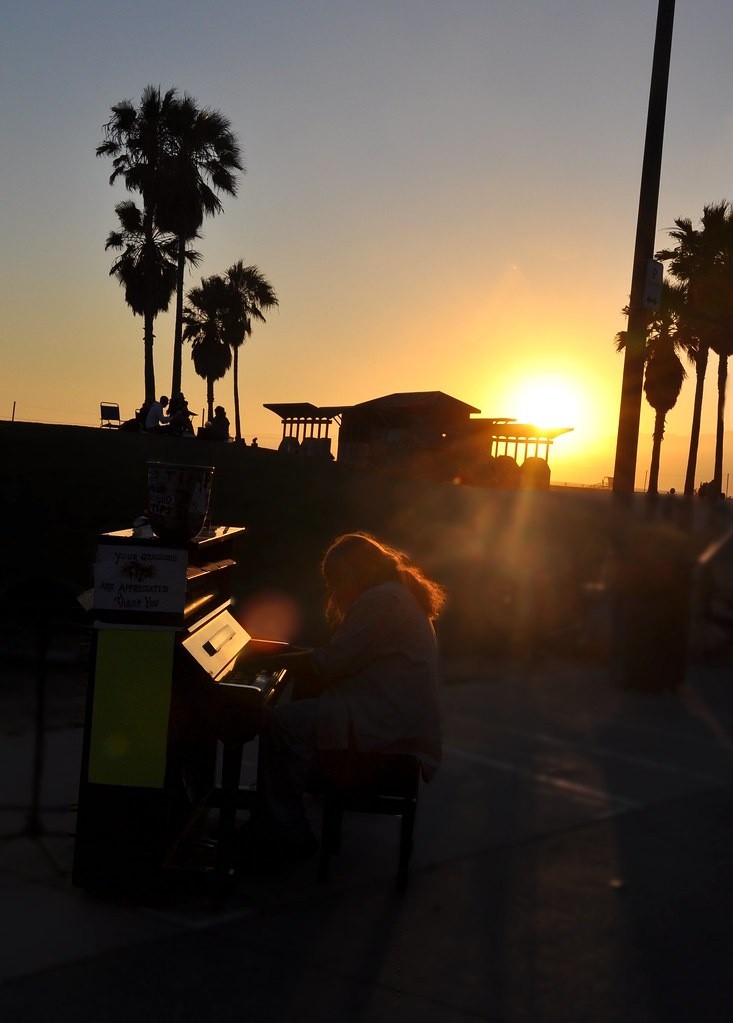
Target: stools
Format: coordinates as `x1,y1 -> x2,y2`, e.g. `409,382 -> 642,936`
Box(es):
321,724 -> 435,888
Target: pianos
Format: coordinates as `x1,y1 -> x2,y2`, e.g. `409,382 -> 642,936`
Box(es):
73,525 -> 298,907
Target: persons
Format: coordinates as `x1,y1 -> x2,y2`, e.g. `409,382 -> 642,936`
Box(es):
241,533 -> 446,888
204,406 -> 229,441
145,393 -> 194,436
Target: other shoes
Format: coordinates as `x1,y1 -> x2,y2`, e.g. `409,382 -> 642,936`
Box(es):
209,813 -> 263,845
284,832 -> 319,871
